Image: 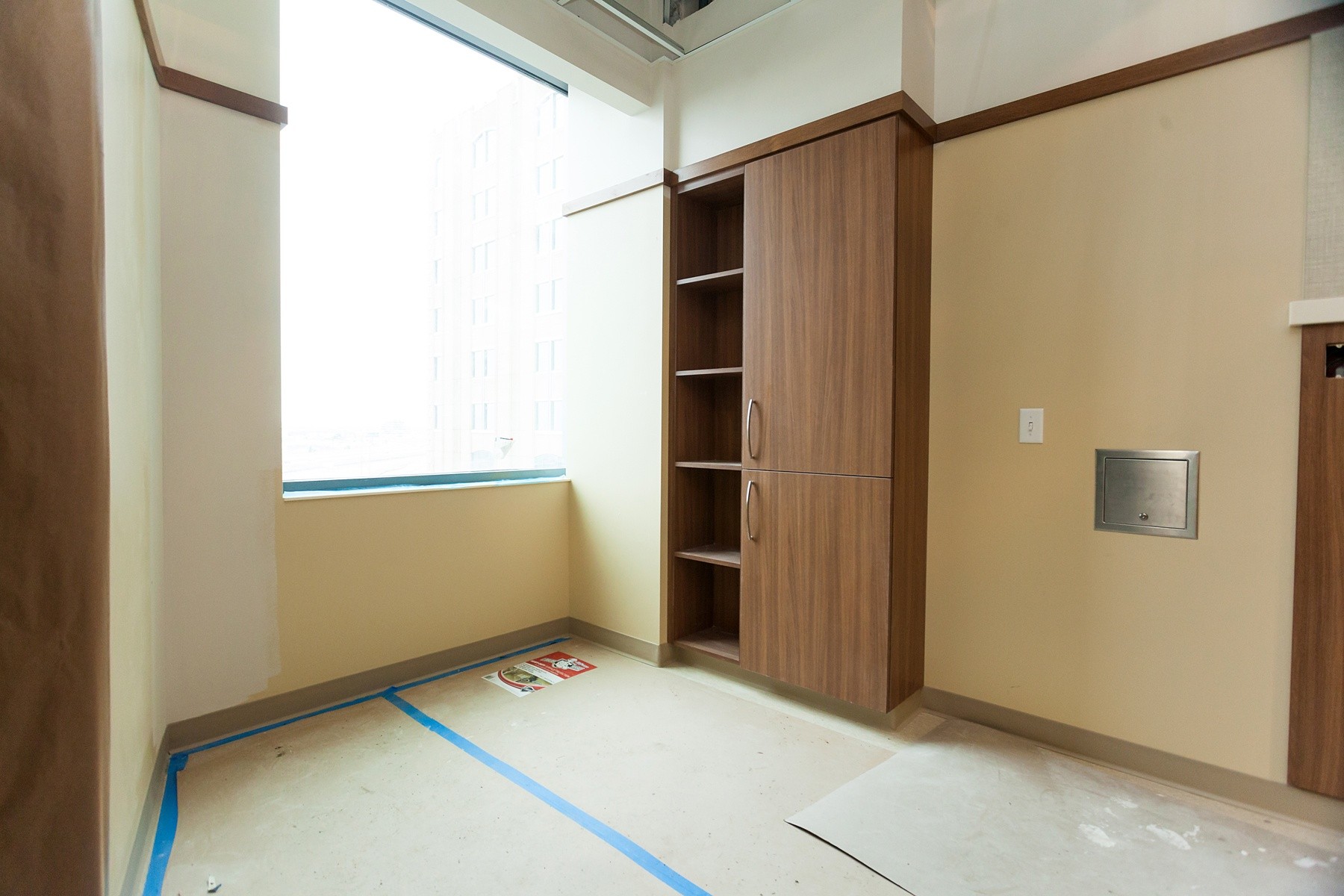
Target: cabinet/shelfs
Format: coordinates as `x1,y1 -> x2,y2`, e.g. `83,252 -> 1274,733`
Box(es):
669,114 -> 935,731
